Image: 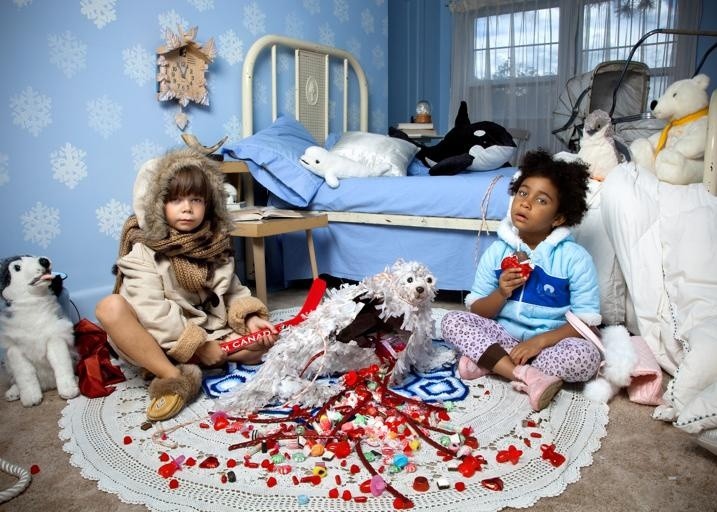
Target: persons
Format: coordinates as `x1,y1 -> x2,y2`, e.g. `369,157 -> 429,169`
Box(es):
440,151 -> 603,413
95,149 -> 280,422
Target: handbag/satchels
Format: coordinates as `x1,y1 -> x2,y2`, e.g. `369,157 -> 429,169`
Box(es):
566,309 -> 665,406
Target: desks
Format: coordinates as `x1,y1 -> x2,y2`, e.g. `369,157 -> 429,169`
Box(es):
409,134 -> 444,148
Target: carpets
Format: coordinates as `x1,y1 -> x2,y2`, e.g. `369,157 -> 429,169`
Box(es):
57,306 -> 610,512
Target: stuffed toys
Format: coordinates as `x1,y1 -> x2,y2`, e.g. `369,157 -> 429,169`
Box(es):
389,101 -> 517,177
576,109 -> 623,184
630,73 -> 710,185
299,146 -> 392,188
0,254 -> 80,407
216,259 -> 456,411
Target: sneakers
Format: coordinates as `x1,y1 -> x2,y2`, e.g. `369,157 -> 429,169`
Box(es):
148,363 -> 202,420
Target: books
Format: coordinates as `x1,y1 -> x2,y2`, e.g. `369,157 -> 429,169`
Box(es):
225,206 -> 305,223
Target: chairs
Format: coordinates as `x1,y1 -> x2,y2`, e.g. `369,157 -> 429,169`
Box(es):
507,128 -> 530,167
184,160 -> 328,308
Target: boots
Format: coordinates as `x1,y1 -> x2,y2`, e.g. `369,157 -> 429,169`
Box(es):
458,355 -> 490,380
511,364 -> 562,410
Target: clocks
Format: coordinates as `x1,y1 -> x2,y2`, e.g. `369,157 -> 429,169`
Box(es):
154,19 -> 230,155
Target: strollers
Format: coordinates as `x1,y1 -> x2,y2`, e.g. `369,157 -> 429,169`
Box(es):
550,28 -> 716,153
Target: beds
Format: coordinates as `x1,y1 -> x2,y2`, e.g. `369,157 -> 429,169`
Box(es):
242,34 -> 717,304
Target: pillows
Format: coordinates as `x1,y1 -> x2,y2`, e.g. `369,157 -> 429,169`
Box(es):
329,130 -> 422,178
220,112 -> 326,208
